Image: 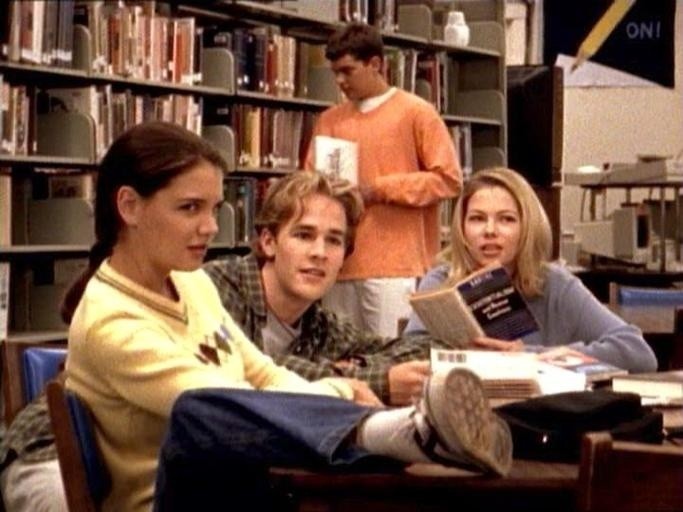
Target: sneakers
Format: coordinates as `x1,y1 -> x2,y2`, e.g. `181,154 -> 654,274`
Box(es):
417,367 -> 515,480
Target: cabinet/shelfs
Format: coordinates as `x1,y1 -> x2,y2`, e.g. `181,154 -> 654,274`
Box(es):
0,0 -> 518,344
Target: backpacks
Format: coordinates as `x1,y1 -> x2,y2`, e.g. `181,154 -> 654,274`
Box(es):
493,387 -> 665,464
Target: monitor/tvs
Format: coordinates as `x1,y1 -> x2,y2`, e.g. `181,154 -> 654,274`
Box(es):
506,65 -> 563,189
574,205 -> 653,264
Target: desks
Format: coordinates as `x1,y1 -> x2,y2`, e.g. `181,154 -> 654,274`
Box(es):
268,365 -> 683,510
571,264 -> 682,289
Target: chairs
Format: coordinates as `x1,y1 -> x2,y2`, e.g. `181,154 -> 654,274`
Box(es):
45,365 -> 111,512
606,280 -> 681,375
576,430 -> 682,510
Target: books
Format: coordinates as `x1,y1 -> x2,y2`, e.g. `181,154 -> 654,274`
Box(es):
611,371 -> 683,429
